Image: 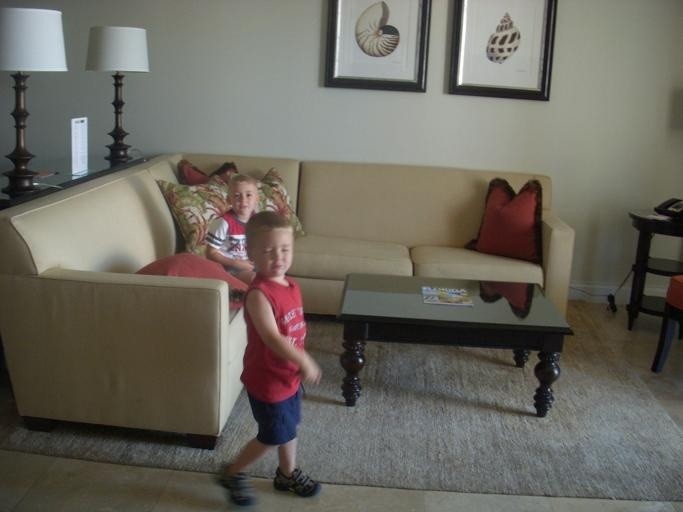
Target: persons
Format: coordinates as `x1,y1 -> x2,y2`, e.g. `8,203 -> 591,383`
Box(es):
207,175 -> 260,286
219,211 -> 322,506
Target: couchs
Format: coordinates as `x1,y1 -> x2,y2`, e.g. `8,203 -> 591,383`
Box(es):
0,150 -> 579,449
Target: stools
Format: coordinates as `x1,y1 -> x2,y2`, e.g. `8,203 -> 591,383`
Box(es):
647,275 -> 682,374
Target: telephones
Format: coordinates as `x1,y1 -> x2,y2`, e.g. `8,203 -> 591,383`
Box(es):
655,197 -> 683,215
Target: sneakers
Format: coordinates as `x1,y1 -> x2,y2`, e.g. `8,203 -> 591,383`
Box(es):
272,464 -> 322,498
211,463 -> 258,507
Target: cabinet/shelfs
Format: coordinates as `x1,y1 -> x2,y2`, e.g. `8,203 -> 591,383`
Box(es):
624,209 -> 683,330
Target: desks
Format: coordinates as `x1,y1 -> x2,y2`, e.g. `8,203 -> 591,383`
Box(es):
1,155 -> 149,210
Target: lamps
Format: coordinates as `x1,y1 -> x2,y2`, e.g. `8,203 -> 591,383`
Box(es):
1,8 -> 68,196
83,25 -> 151,165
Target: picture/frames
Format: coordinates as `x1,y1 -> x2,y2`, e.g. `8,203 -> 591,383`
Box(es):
321,0 -> 434,94
440,1 -> 558,106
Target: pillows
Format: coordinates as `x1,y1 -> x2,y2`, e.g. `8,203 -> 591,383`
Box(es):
157,176 -> 233,254
173,159 -> 238,187
466,175 -> 544,266
133,254 -> 252,298
244,165 -> 305,239
478,282 -> 536,320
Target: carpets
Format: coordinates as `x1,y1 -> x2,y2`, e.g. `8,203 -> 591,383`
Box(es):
0,311 -> 683,499
